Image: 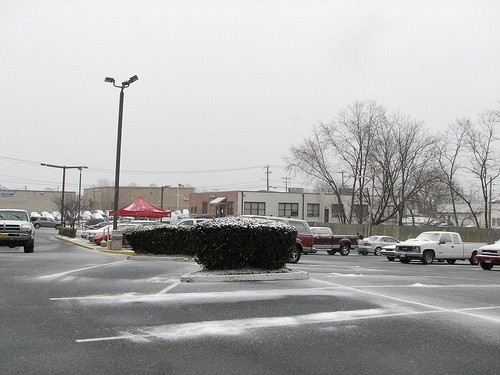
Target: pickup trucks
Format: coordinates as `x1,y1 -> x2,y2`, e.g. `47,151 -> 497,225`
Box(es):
379,230 -> 481,265
309,226 -> 360,257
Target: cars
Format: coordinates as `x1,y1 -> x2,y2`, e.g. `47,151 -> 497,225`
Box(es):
29,209 -> 211,246
357,235 -> 401,256
475,238 -> 500,271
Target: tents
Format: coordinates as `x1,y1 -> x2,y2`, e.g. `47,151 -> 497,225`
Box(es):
109,198 -> 171,223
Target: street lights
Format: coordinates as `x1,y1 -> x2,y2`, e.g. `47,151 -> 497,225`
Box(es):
104,74 -> 140,231
78,167 -> 83,227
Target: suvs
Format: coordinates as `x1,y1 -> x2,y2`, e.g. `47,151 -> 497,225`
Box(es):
238,214 -> 316,264
0,208 -> 35,253
30,216 -> 61,230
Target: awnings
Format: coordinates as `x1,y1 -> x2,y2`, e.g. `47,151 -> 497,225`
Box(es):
210,197 -> 226,206
